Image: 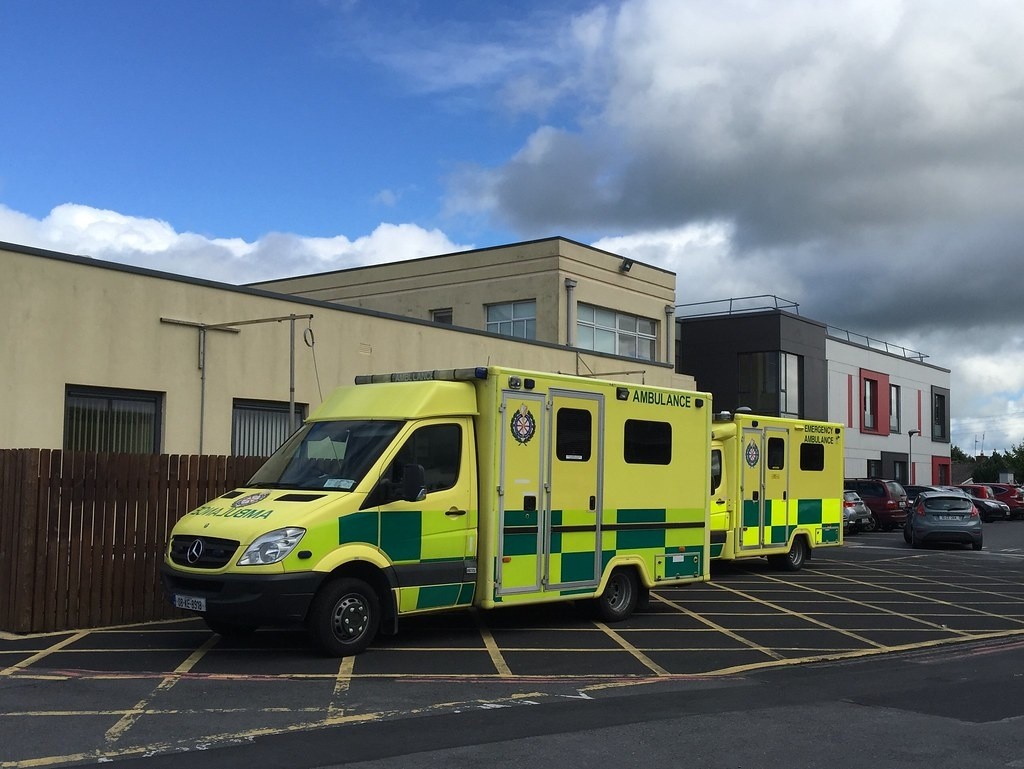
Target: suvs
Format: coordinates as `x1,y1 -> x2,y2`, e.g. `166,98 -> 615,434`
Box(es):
844,477 -> 910,532
902,483 -> 1024,523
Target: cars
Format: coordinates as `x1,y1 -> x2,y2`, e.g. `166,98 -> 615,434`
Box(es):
843,490 -> 871,534
904,492 -> 983,551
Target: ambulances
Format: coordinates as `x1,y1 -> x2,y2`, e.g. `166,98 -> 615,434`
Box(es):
161,366 -> 713,656
712,413 -> 845,570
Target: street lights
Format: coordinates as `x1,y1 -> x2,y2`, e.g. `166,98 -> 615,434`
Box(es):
908,428 -> 921,485
